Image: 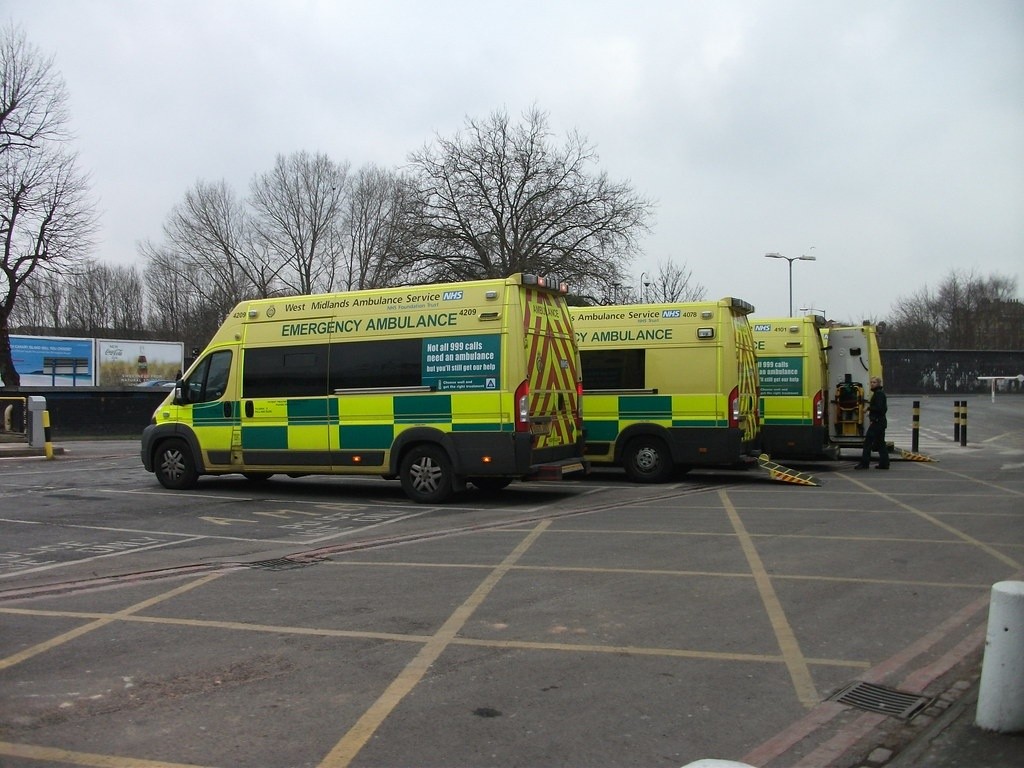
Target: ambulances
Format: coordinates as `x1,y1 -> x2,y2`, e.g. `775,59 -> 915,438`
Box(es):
564,297 -> 761,483
140,273 -> 586,504
818,326 -> 830,363
747,316 -> 884,458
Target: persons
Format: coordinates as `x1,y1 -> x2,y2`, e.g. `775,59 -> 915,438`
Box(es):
854,376 -> 890,470
206,357 -> 231,398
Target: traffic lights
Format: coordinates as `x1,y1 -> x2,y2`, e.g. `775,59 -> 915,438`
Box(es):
191,347 -> 199,362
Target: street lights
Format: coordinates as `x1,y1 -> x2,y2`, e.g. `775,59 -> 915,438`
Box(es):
765,252 -> 817,317
640,272 -> 650,304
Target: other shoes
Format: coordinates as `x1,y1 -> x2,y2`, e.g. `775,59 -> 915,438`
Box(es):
875,464 -> 890,469
854,462 -> 869,470
